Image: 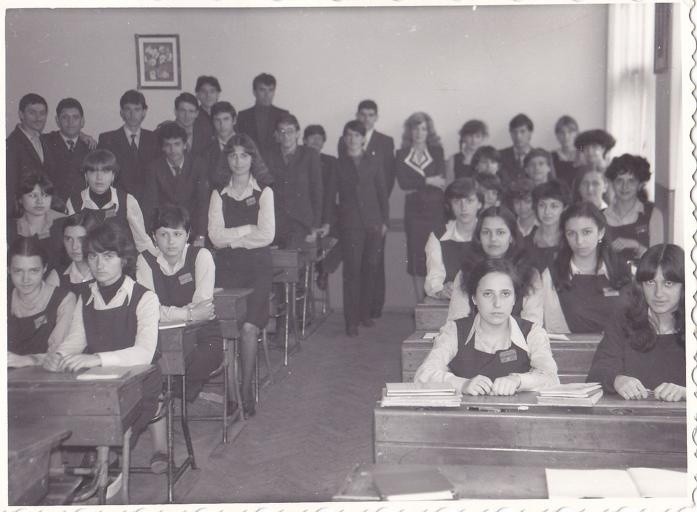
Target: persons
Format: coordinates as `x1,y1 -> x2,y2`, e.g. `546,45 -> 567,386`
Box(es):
410,257 -> 560,395
7,235 -> 77,370
7,72 -> 681,336
42,223 -> 161,497
206,135 -> 274,421
583,243 -> 684,401
132,203 -> 218,472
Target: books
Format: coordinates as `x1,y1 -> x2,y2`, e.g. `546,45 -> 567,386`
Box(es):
368,469 -> 460,502
545,465 -> 687,498
421,328 -> 570,343
537,382 -> 604,409
75,367 -> 132,382
377,381 -> 462,406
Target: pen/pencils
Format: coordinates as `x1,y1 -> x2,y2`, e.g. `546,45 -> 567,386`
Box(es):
467,406 -> 529,412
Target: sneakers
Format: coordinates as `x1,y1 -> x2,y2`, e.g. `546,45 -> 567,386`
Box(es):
151,452 -> 171,474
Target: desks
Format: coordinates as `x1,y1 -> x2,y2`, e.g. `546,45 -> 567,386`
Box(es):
173,286 -> 255,424
7,363 -> 164,504
9,426 -> 75,506
268,230 -> 341,369
158,315 -> 225,503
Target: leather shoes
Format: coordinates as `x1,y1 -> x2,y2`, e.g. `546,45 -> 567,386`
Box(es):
315,260 -> 328,290
225,399 -> 237,417
237,387 -> 255,420
346,308 -> 381,338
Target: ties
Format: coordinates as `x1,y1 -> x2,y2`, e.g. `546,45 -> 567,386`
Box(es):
173,166 -> 181,175
32,136 -> 42,154
131,134 -> 138,152
68,140 -> 74,152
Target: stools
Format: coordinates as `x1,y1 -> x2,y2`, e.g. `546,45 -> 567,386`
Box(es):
60,337 -> 244,503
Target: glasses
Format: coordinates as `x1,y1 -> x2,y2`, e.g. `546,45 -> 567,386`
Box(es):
279,129 -> 296,136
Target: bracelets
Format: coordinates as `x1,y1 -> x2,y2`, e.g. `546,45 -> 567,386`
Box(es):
187,307 -> 194,322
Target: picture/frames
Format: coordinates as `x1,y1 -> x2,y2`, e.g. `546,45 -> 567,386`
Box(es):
134,33 -> 182,91
